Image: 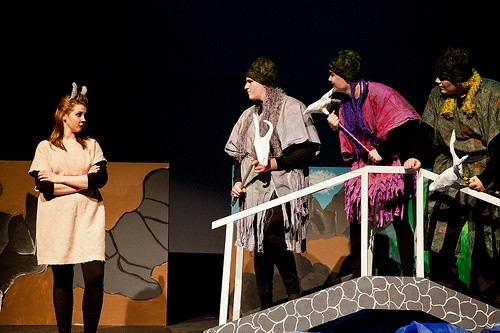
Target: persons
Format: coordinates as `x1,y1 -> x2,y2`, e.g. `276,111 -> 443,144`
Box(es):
224,58 -> 321,310
326,49 -> 423,279
421,48 -> 500,307
28,83 -> 108,333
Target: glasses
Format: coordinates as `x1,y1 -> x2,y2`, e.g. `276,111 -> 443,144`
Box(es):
433,71 -> 456,81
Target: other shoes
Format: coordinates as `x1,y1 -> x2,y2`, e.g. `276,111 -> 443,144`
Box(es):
288,294 -> 300,301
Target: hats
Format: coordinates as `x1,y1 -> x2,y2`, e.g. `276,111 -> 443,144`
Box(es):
328,50 -> 362,82
246,57 -> 280,89
435,48 -> 473,83
